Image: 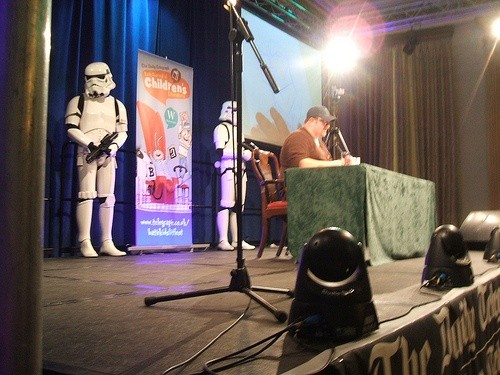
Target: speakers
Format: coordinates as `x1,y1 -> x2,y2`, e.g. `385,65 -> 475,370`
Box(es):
459,209 -> 500,251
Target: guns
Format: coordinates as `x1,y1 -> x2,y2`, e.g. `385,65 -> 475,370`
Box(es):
85,133 -> 118,163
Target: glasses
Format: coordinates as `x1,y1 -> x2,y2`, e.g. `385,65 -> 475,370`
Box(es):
314,116 -> 328,128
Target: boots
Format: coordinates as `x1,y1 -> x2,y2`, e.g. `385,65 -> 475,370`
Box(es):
99,194 -> 127,256
229,212 -> 255,249
75,199 -> 98,257
217,209 -> 235,250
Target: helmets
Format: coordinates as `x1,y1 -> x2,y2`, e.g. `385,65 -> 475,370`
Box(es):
84,61 -> 116,98
219,100 -> 238,126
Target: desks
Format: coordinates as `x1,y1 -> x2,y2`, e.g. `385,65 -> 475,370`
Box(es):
284,162 -> 436,265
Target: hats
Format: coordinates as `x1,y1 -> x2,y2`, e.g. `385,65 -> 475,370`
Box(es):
307,106 -> 336,122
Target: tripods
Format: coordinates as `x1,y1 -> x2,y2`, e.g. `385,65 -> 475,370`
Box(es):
144,0 -> 295,324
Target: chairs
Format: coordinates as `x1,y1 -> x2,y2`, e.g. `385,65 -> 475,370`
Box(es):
250,148 -> 295,259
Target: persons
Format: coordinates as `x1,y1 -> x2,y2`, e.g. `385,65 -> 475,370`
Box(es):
65,62 -> 128,257
277,105 -> 353,201
213,101 -> 255,250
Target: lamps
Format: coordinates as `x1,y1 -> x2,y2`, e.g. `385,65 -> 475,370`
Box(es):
419,224 -> 473,293
402,31 -> 419,54
278,227 -> 378,348
